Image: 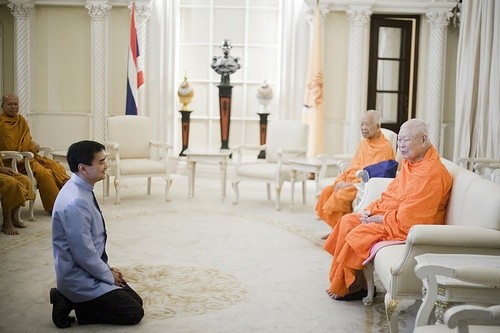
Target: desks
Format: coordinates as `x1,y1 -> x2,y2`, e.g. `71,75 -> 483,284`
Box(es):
182,147 -> 233,202
49,150 -> 111,205
287,155 -> 347,220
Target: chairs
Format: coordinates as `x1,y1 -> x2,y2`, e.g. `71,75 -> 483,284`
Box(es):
377,128 -> 397,154
0,115 -> 500,333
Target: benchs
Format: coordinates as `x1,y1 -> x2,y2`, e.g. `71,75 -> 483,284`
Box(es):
351,156 -> 500,333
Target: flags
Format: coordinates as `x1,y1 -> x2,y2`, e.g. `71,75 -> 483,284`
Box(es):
124,1 -> 145,115
303,8 -> 325,157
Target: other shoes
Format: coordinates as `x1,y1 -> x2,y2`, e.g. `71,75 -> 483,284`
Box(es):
49,287 -> 76,329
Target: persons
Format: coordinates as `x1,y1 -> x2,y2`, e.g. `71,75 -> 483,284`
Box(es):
50,139 -> 145,328
315,110 -> 395,241
0,167 -> 36,235
323,119 -> 452,301
0,95 -> 71,215
